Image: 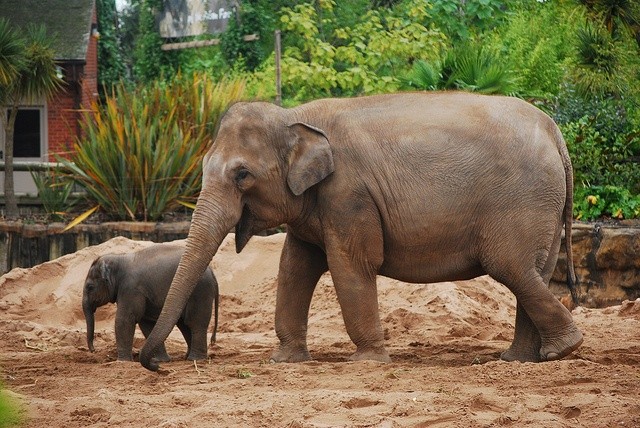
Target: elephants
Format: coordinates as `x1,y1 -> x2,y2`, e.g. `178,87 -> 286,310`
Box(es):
139,91 -> 584,371
82,242 -> 218,361
